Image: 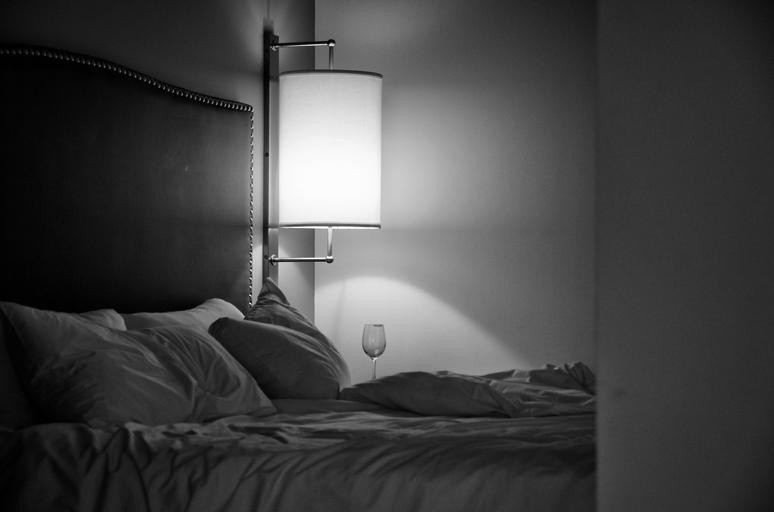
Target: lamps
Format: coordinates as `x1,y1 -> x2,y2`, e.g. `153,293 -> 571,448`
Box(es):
263,34 -> 385,267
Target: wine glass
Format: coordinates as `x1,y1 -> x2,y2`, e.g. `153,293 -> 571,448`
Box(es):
363,323 -> 386,381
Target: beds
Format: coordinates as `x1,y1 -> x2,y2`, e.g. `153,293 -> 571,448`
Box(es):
0,49 -> 596,512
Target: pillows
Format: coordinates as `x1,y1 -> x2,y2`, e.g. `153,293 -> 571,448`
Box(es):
56,311 -> 279,429
149,297 -> 248,343
207,277 -> 348,408
2,298 -> 130,395
